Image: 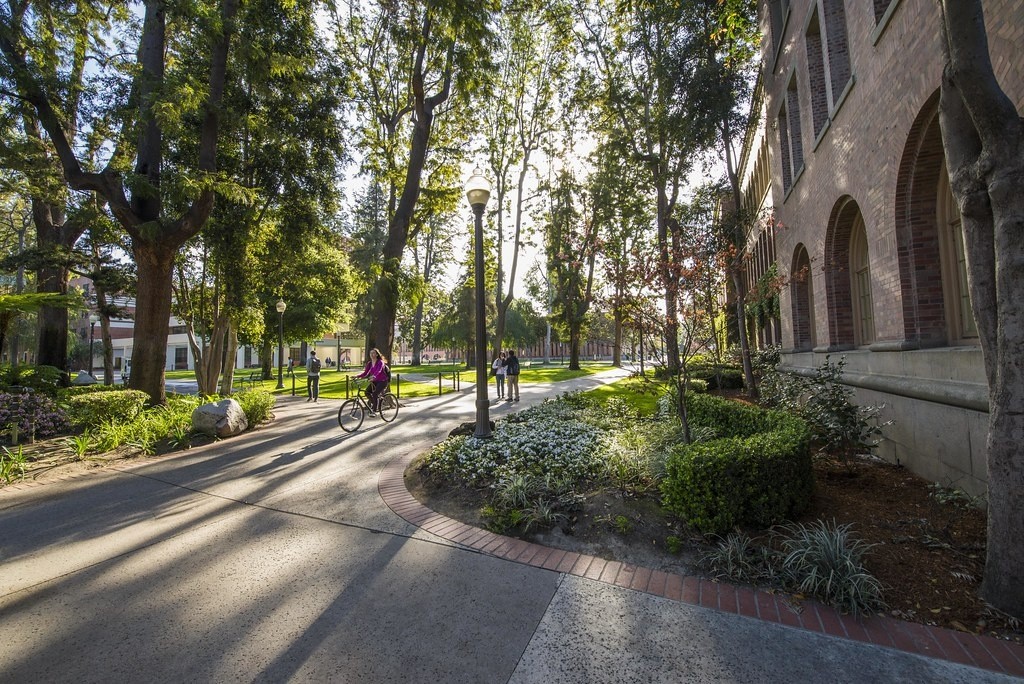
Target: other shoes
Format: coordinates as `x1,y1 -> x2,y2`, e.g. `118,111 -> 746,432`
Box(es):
497,396 -> 499,399
502,396 -> 505,398
307,398 -> 312,402
369,412 -> 376,417
314,399 -> 317,402
514,398 -> 519,401
505,398 -> 512,402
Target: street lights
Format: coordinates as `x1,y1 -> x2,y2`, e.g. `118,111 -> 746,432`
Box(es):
452,336 -> 456,366
275,296 -> 286,389
463,161 -> 496,440
87,314 -> 97,377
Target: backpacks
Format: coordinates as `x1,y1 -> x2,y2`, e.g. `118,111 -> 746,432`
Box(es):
384,365 -> 391,383
508,358 -> 519,375
310,357 -> 321,373
291,361 -> 294,366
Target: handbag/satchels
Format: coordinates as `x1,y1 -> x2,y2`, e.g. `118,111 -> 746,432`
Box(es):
491,369 -> 498,376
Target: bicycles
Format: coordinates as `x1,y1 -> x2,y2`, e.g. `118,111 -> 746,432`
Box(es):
337,377 -> 399,432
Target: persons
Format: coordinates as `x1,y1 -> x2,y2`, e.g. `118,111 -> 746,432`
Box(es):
492,350 -> 519,401
286,357 -> 294,377
326,358 -> 331,367
351,348 -> 389,416
306,351 -> 321,402
